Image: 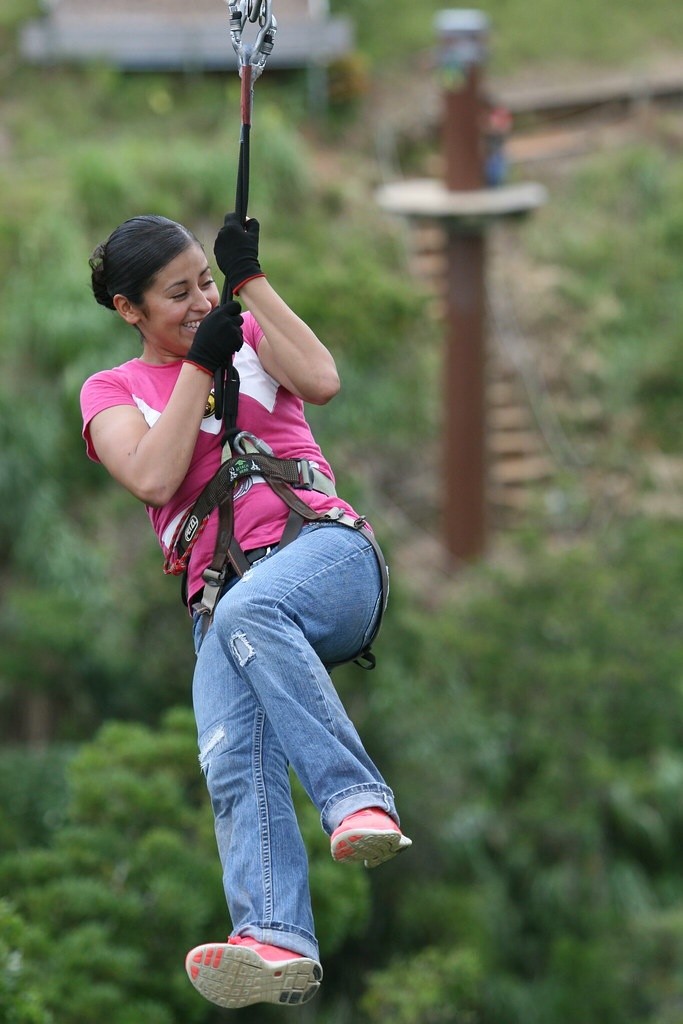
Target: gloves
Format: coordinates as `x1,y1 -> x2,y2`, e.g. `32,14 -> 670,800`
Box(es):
213,213 -> 268,295
182,300 -> 244,378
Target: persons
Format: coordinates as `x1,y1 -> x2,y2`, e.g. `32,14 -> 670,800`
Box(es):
75,214 -> 409,1010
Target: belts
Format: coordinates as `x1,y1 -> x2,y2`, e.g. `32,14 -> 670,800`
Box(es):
223,546 -> 267,583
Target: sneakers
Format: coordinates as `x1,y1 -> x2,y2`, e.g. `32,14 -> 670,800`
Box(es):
331,807 -> 414,870
185,935 -> 324,1009
